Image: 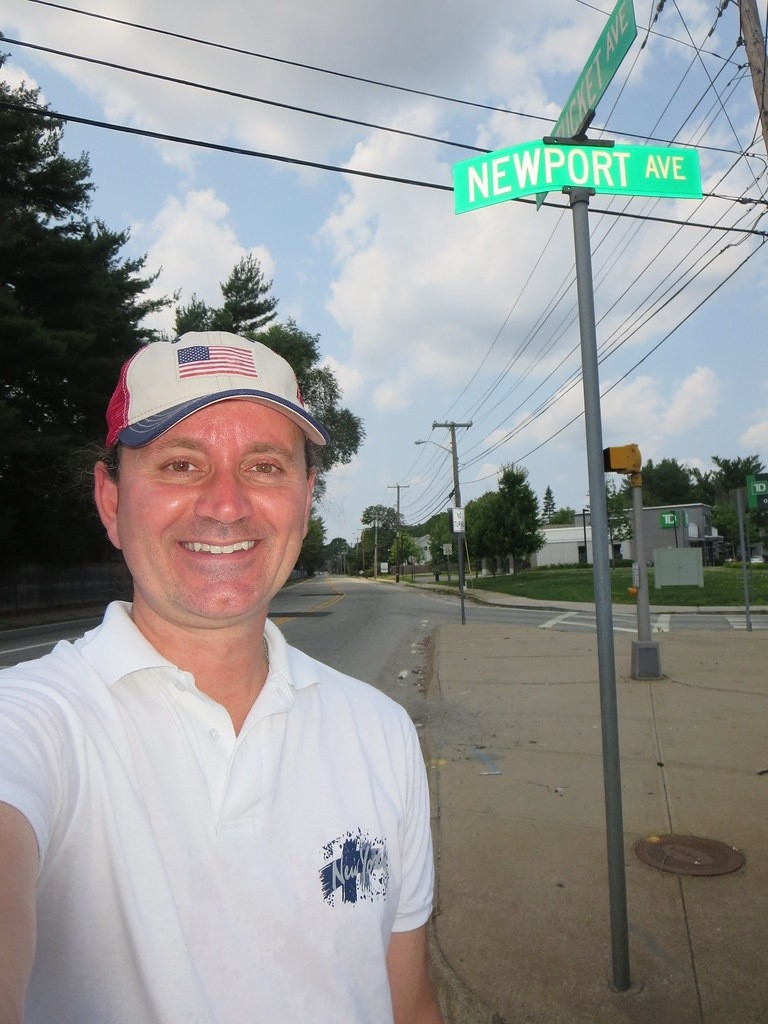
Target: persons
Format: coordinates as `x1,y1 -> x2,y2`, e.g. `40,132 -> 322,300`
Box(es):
0,330 -> 437,1024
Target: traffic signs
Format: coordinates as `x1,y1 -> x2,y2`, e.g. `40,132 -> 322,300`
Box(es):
451,146 -> 709,215
526,0 -> 639,210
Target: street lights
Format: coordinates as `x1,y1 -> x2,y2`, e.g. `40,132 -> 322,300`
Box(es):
414,438 -> 468,588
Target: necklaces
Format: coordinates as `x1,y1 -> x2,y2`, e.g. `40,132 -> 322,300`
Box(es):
262,638 -> 270,677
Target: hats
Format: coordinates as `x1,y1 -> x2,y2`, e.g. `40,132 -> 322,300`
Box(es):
104,333 -> 328,452
